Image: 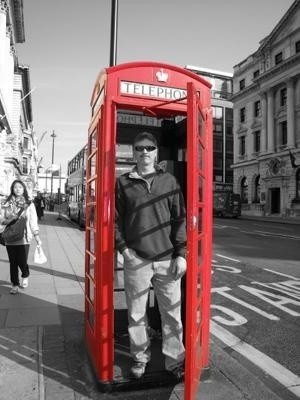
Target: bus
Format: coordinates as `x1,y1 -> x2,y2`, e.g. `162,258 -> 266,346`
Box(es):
66,142 -> 136,228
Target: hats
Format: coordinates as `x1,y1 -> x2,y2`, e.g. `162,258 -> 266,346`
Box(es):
133,132 -> 156,142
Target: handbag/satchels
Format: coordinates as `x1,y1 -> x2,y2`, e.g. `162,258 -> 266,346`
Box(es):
1,218 -> 26,246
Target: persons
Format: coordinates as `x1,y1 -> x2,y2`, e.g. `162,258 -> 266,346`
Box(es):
35,192 -> 45,221
0,180 -> 41,294
113,130 -> 188,381
33,190 -> 41,202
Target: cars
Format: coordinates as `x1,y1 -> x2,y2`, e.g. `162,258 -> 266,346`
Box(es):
213,183 -> 242,219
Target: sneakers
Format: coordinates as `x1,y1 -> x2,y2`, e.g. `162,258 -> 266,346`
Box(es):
10,285 -> 18,294
22,277 -> 28,288
130,360 -> 146,378
168,365 -> 185,379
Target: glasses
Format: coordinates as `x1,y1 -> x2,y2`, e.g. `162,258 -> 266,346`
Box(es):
134,146 -> 159,151
